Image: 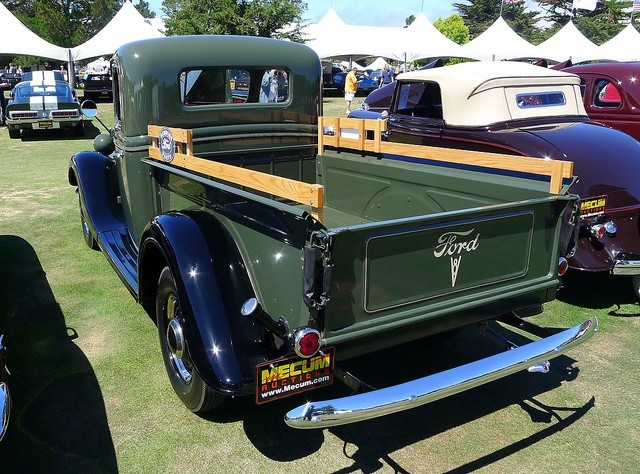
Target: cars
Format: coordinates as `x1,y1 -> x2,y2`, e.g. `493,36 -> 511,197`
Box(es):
345,61 -> 640,295
332,71 -> 378,97
0,72 -> 22,88
548,60 -> 639,144
81,73 -> 113,102
5,70 -> 85,139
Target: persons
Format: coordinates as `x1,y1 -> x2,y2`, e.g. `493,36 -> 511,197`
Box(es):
345,68 -> 357,114
378,64 -> 392,89
395,64 -> 401,73
8,64 -> 24,76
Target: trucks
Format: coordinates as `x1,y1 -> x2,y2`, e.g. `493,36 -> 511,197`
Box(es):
321,60 -> 334,97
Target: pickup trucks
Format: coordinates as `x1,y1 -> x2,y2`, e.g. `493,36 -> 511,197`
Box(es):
67,34 -> 600,431
